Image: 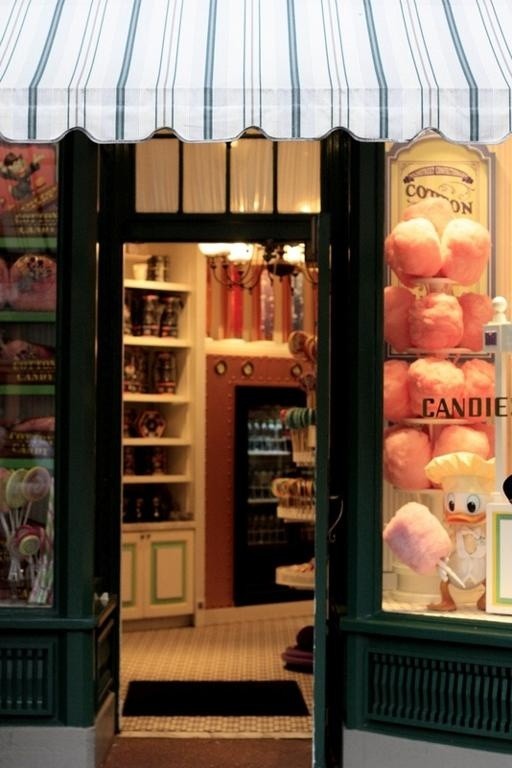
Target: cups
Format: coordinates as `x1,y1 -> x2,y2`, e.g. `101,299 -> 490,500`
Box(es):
132,262 -> 147,281
149,254 -> 167,282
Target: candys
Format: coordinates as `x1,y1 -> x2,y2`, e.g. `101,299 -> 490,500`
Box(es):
271,331 -> 318,513
383,501 -> 455,579
0,466 -> 51,557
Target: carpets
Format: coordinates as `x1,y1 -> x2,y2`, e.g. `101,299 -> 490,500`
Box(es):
123,681 -> 309,717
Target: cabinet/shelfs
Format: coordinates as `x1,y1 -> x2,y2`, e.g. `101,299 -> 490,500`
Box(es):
382,154 -> 512,624
270,262 -> 325,672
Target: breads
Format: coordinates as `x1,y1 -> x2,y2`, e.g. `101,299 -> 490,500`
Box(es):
0,253 -> 56,435
383,216 -> 497,491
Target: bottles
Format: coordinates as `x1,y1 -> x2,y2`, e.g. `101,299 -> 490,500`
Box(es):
123,292 -> 181,394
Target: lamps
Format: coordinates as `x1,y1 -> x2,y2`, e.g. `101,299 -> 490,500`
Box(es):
194,244 -> 331,288
